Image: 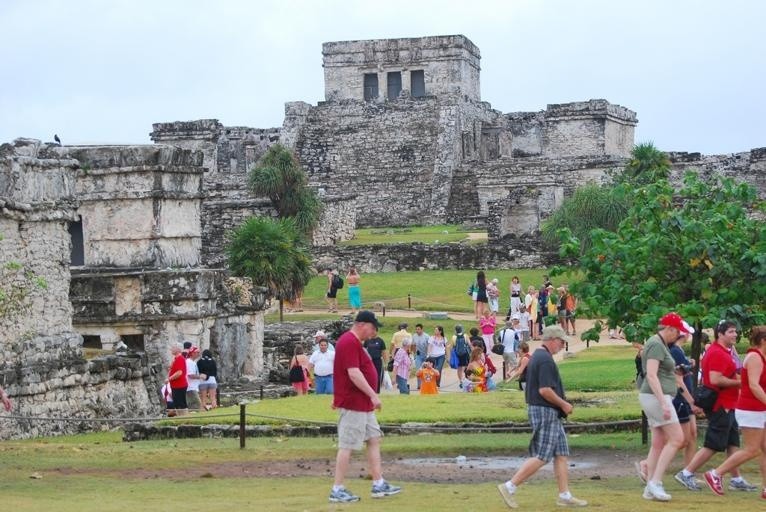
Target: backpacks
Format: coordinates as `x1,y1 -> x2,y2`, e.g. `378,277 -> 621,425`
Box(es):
387,358 -> 395,372
455,335 -> 468,356
332,275 -> 344,290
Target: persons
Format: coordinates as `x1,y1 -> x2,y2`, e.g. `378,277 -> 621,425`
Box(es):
167,343 -> 189,409
290,316 -> 532,394
634,311 -> 766,501
328,311 -> 401,503
185,346 -> 207,408
326,267 -> 339,313
497,325 -> 588,508
197,349 -> 218,408
468,270 -> 580,336
346,269 -> 361,313
180,342 -> 193,357
161,382 -> 175,417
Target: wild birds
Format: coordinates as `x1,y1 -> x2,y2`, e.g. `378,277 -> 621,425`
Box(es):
54,134 -> 61,144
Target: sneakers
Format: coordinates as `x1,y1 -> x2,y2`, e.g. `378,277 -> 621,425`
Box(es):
674,471 -> 702,492
329,488 -> 362,503
285,307 -> 305,313
370,483 -> 401,499
497,483 -> 517,508
704,471 -> 723,495
634,461 -> 647,484
327,305 -> 338,315
556,496 -> 589,507
726,479 -> 758,491
642,484 -> 673,500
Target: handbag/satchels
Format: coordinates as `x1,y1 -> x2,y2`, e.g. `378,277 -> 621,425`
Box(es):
490,344 -> 505,355
696,383 -> 720,413
288,355 -> 305,384
672,389 -> 690,423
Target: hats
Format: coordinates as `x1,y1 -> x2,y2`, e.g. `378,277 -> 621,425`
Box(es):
455,324 -> 463,333
545,283 -> 566,294
398,321 -> 408,329
542,325 -> 572,344
312,329 -> 325,339
660,314 -> 694,336
355,310 -> 383,329
